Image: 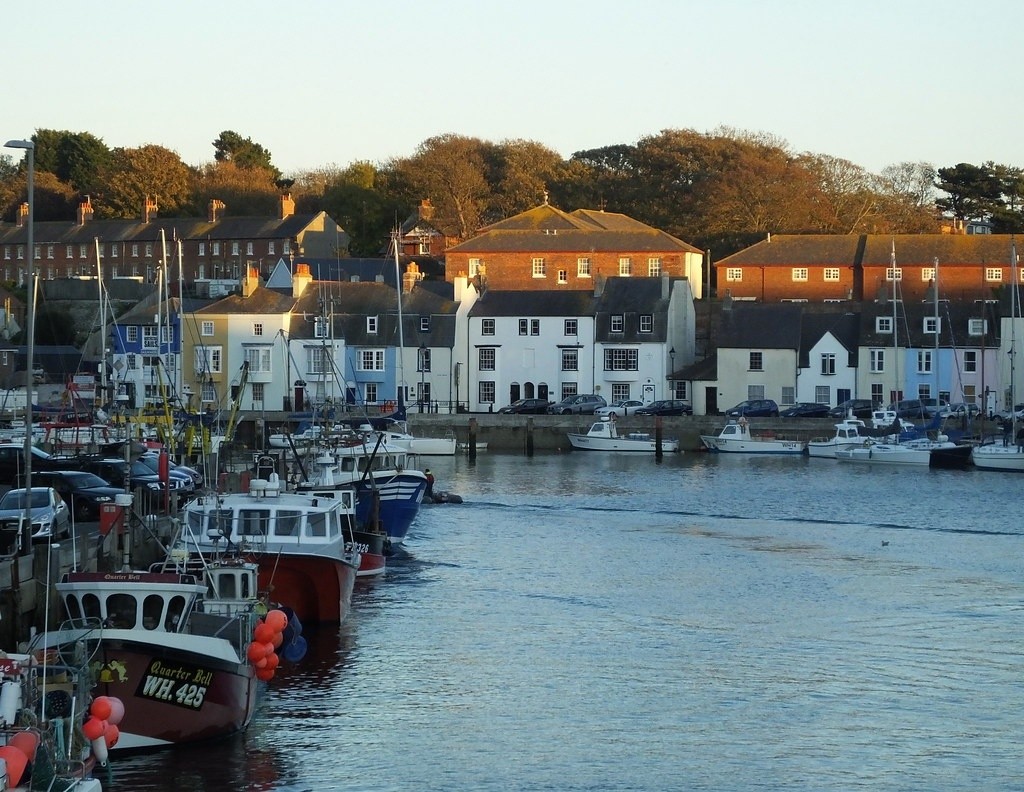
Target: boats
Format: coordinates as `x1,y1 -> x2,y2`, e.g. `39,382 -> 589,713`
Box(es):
459,441 -> 488,448
424,492 -> 462,504
699,418 -> 806,454
808,419 -> 894,459
566,416 -> 679,453
871,410 -> 915,432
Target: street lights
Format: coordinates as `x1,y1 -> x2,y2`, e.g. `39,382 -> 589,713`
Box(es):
5,140 -> 34,552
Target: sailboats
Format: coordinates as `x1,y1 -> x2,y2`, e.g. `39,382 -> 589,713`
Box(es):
972,239 -> 1024,470
835,238 -> 974,467
0,226 -> 456,792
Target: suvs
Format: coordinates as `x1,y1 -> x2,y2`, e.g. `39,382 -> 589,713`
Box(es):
79,459 -> 187,508
547,394 -> 607,416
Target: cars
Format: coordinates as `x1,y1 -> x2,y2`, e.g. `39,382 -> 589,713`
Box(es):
919,398 -> 950,418
137,456 -> 195,495
593,399 -> 647,417
726,400 -> 780,418
634,400 -> 693,417
0,443 -> 80,485
143,451 -> 203,490
872,400 -> 929,420
930,403 -> 979,420
498,398 -> 553,415
987,403 -> 1024,424
0,488 -> 71,544
824,399 -> 882,419
778,403 -> 830,418
14,470 -> 134,519
